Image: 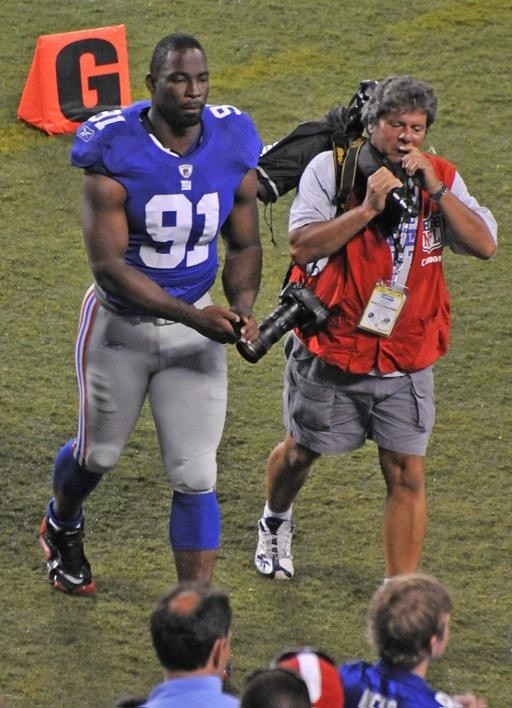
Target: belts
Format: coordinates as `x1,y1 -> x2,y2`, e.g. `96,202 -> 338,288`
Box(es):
92,283 -> 176,326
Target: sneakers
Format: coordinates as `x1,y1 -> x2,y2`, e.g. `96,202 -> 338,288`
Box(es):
39,497 -> 96,596
253,516 -> 297,581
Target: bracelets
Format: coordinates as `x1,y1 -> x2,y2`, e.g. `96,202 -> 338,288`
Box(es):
429,181 -> 448,204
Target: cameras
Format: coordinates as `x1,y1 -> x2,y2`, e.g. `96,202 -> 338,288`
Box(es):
233,278 -> 332,365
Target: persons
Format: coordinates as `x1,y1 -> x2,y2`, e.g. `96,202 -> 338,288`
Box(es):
238,667 -> 311,708
337,573 -> 494,708
33,33 -> 266,597
249,70 -> 497,586
117,580 -> 240,708
